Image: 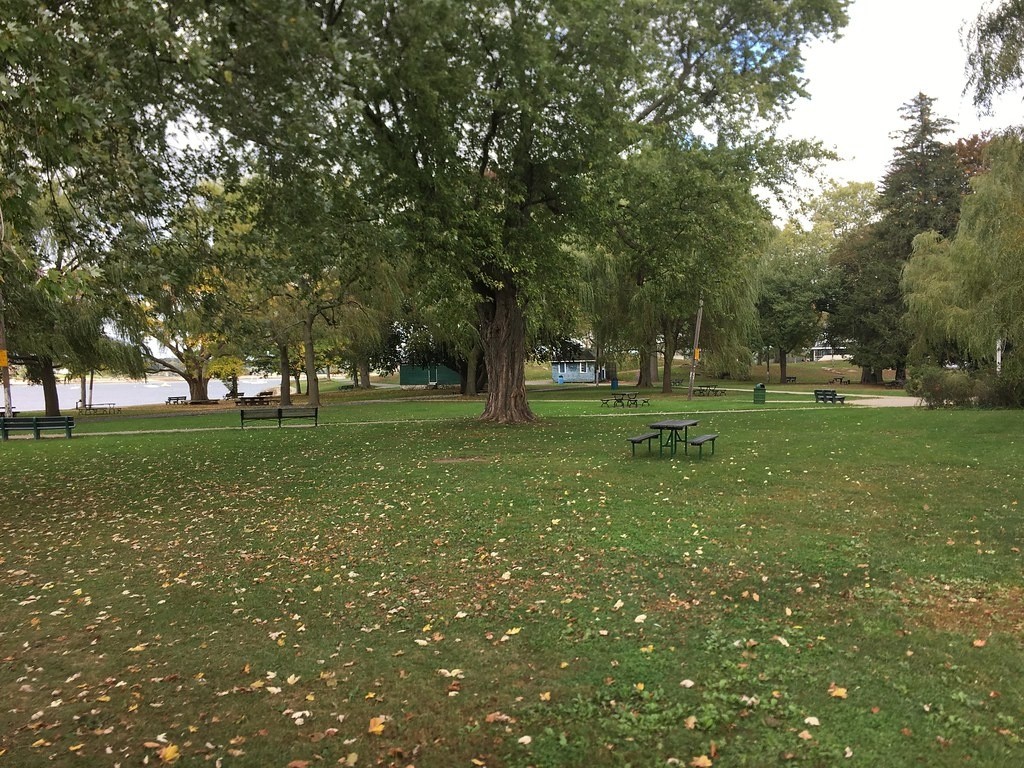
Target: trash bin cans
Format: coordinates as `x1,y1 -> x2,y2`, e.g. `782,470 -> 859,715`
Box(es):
753,383 -> 766,404
558,375 -> 563,384
611,377 -> 618,390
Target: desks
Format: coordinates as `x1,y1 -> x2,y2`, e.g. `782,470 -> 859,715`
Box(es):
834,376 -> 844,384
650,419 -> 700,458
611,391 -> 640,408
83,403 -> 116,415
239,397 -> 271,406
698,385 -> 718,397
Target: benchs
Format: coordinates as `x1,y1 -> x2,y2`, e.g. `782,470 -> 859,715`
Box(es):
338,385 -> 354,391
629,398 -> 651,407
165,396 -> 187,405
814,389 -> 846,404
686,435 -> 719,460
626,432 -> 661,456
225,393 -> 245,401
240,407 -> 320,430
0,416 -> 76,441
0,407 -> 19,417
693,388 -> 726,396
235,400 -> 269,406
78,408 -> 122,415
843,380 -> 850,385
257,392 -> 273,397
827,379 -> 834,384
600,399 -> 628,407
